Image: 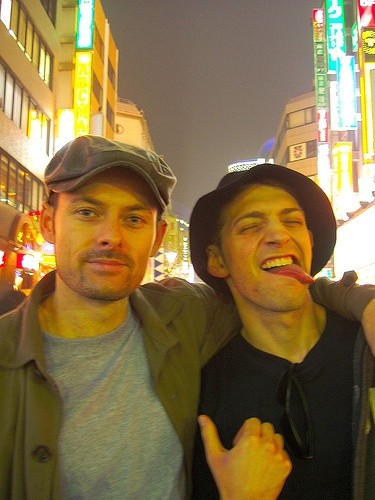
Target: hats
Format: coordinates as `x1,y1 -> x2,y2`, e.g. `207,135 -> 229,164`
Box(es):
189,163 -> 337,296
44,135 -> 177,211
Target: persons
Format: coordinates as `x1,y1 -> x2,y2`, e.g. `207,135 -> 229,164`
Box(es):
189,163 -> 375,500
0,133 -> 375,500
